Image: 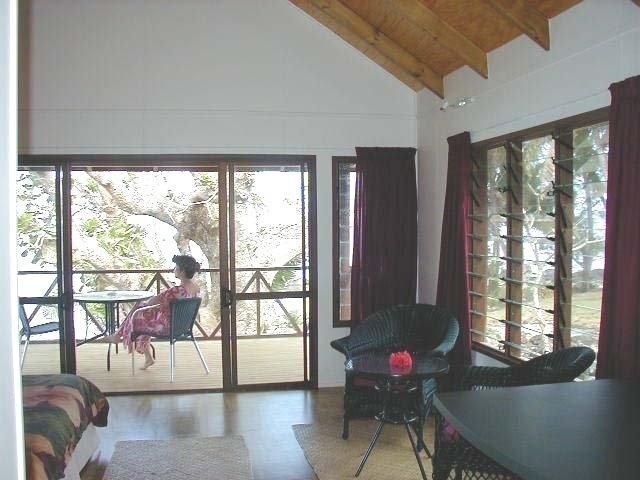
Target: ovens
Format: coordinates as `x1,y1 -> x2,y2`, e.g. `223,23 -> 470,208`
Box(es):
22,374 -> 109,480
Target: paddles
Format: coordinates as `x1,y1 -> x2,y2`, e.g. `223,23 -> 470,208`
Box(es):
292,407 -> 519,480
99,434 -> 254,480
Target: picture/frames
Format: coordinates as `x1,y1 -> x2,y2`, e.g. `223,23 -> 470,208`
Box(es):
436,375 -> 639,480
72,290 -> 154,373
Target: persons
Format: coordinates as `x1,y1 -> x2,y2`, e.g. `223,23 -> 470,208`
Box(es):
102,254 -> 201,370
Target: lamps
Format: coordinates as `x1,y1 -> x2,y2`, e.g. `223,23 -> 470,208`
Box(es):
330,300 -> 460,452
431,347 -> 596,472
131,298 -> 210,377
19,303 -> 60,371
